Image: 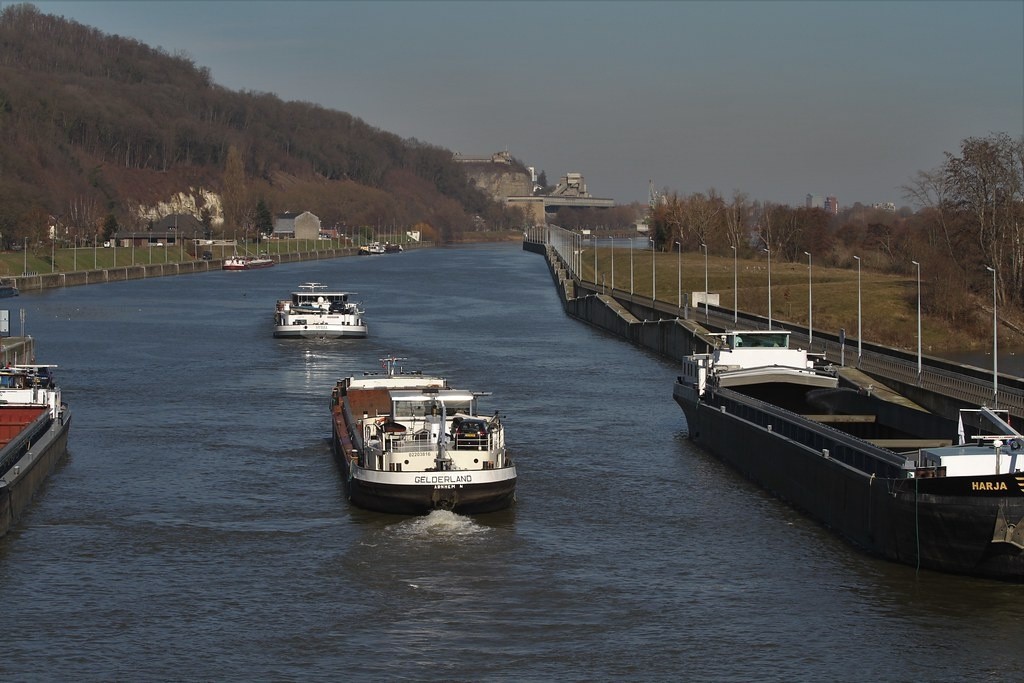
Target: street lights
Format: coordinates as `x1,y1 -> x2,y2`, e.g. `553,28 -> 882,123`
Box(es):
650,240 -> 655,309
592,235 -> 597,291
676,242 -> 681,307
853,256 -> 862,369
608,236 -> 613,297
764,249 -> 772,331
628,238 -> 633,301
731,245 -> 737,330
114,233 -> 118,267
805,252 -> 812,351
74,235 -> 78,272
132,233 -> 136,267
702,244 -> 708,324
912,261 -> 922,386
95,234 -> 98,270
987,267 -> 997,408
24,237 -> 28,276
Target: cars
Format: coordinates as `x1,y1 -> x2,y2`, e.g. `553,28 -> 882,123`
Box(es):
321,233 -> 331,238
104,241 -> 111,248
157,241 -> 163,246
203,251 -> 212,260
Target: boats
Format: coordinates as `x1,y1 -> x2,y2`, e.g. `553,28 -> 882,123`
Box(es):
672,329 -> 1024,573
222,245 -> 275,270
0,309 -> 73,540
272,283 -> 369,337
359,241 -> 404,254
328,353 -> 518,514
